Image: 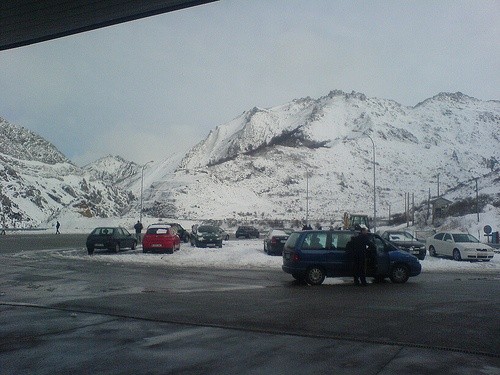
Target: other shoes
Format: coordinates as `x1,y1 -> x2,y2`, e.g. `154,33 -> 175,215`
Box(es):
360,284 -> 367,286
354,283 -> 360,286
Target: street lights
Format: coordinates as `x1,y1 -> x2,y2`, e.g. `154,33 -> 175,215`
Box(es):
140,160 -> 154,223
352,129 -> 376,232
293,161 -> 309,223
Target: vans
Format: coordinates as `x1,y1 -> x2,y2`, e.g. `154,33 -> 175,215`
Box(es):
282,229 -> 422,285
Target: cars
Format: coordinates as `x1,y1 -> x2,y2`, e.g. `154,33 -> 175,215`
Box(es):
86,226 -> 138,253
263,228 -> 296,255
236,226 -> 260,238
217,227 -> 230,241
380,230 -> 427,260
156,223 -> 189,243
142,225 -> 181,253
426,231 -> 495,261
189,224 -> 223,248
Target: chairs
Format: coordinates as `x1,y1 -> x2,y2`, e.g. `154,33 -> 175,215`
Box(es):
331,239 -> 335,247
313,238 -> 323,248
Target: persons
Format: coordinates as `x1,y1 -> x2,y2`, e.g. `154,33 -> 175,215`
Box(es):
0,221 -> 6,235
56,221 -> 60,234
134,220 -> 143,243
350,228 -> 375,286
